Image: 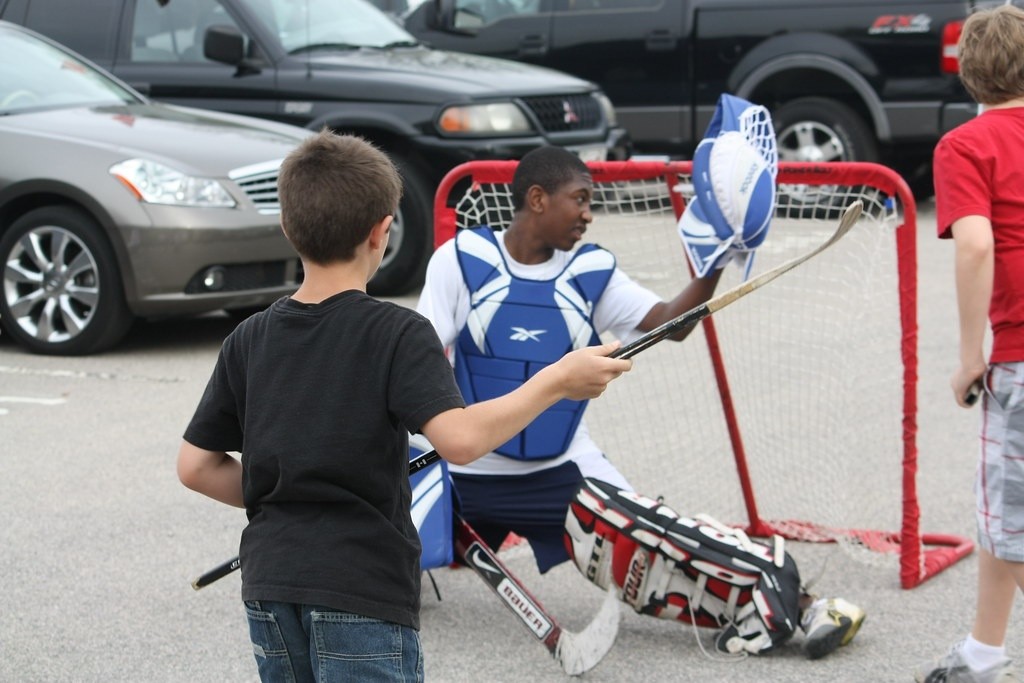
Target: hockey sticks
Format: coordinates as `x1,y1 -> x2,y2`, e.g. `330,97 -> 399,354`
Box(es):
191,198 -> 864,592
449,511 -> 621,676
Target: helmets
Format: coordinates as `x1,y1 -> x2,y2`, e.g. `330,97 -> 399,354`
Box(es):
679,95 -> 776,279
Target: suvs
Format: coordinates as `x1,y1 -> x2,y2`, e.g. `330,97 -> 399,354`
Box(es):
400,1 -> 1023,219
0,1 -> 633,299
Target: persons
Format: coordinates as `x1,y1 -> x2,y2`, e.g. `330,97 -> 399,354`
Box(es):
177,127 -> 866,683
909,5 -> 1024,683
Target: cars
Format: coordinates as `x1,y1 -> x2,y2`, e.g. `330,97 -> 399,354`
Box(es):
0,17 -> 404,355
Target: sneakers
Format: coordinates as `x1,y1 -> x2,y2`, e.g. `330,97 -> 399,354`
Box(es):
801,597 -> 868,660
914,640 -> 1011,682
